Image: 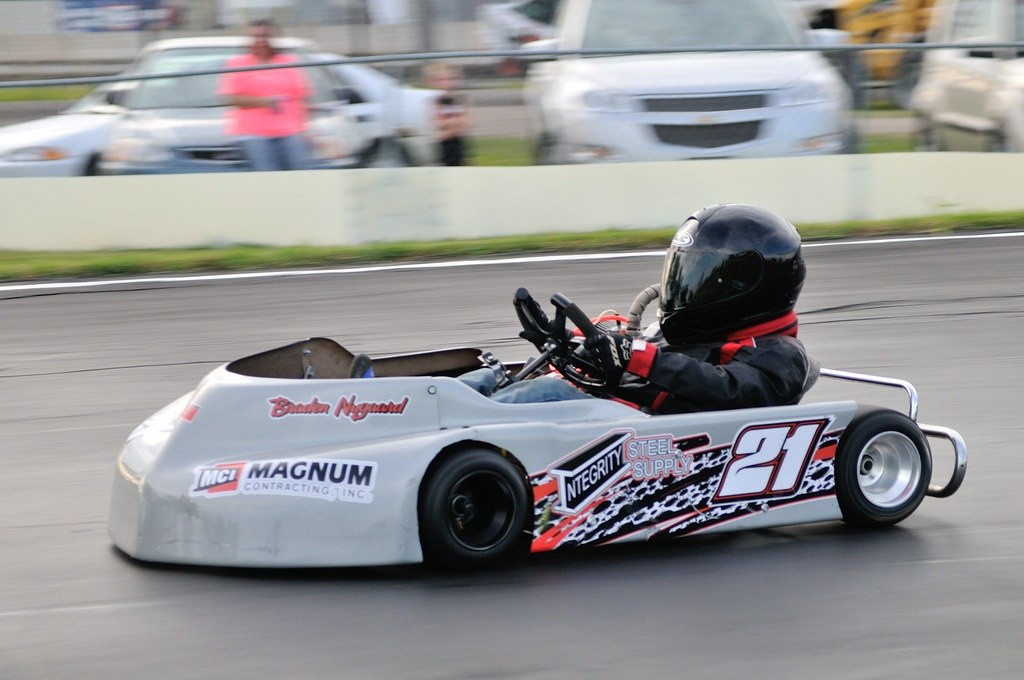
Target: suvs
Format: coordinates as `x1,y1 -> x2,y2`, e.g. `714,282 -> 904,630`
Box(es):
513,1 -> 856,165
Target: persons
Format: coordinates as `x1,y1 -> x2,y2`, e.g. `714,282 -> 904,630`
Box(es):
431,61 -> 474,166
215,19 -> 315,171
348,203 -> 807,416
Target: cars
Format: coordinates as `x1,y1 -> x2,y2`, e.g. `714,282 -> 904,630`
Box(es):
0,45 -> 472,176
910,2 -> 1023,152
97,33 -> 364,170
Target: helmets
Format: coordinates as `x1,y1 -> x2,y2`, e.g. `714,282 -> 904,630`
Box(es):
659,204 -> 806,346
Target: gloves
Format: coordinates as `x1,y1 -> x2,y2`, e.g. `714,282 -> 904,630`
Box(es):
549,320 -> 574,340
584,334 -> 633,372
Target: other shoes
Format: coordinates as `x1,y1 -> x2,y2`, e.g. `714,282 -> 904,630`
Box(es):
350,354 -> 375,378
